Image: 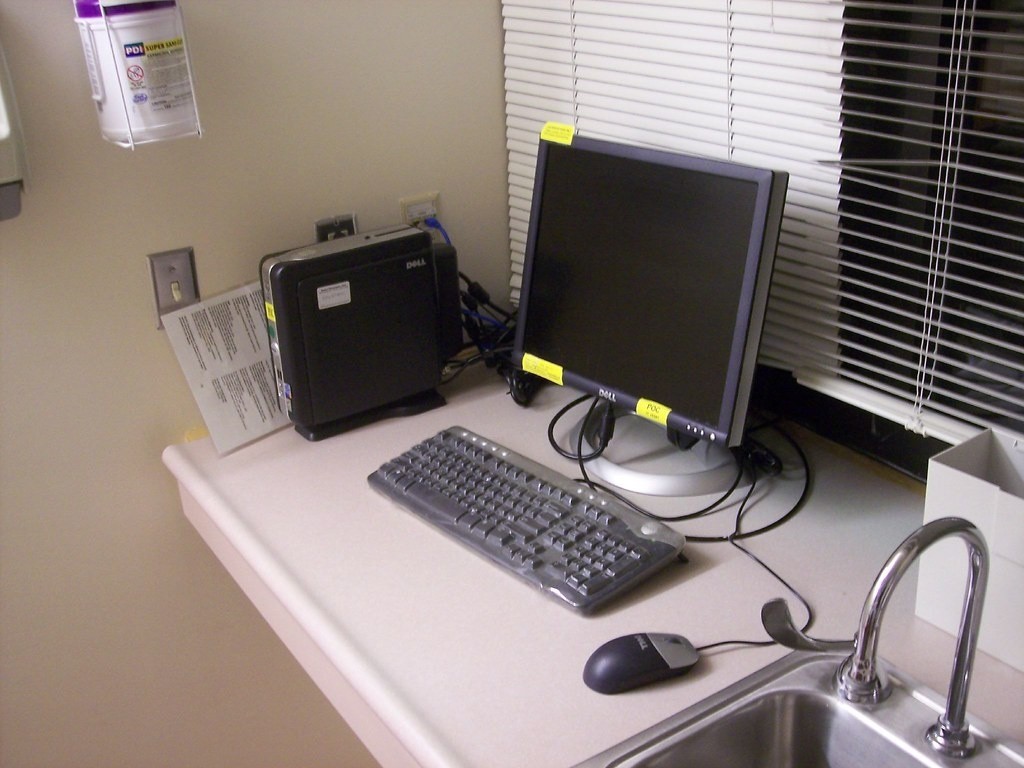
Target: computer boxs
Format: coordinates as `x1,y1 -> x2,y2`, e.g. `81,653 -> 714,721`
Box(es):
258,223 -> 443,427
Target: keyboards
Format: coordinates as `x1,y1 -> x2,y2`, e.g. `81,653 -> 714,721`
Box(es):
366,425 -> 687,614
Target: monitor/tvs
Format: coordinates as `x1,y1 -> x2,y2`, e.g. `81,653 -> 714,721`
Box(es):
509,128 -> 790,497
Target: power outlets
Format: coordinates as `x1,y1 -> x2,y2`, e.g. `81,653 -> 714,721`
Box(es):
314,212 -> 356,243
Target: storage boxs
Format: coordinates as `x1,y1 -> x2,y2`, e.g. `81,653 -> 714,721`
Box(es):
915,428 -> 1023,672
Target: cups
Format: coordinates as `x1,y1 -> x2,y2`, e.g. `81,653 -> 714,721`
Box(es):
73,0 -> 199,142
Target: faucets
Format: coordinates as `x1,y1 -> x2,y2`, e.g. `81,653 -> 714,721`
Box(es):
834,517 -> 989,758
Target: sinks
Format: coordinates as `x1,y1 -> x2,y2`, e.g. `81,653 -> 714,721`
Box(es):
575,641 -> 1024,768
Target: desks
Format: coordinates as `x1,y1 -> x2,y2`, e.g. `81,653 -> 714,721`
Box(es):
161,357 -> 927,768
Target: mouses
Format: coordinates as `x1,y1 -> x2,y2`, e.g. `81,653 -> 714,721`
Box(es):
582,633 -> 699,696
